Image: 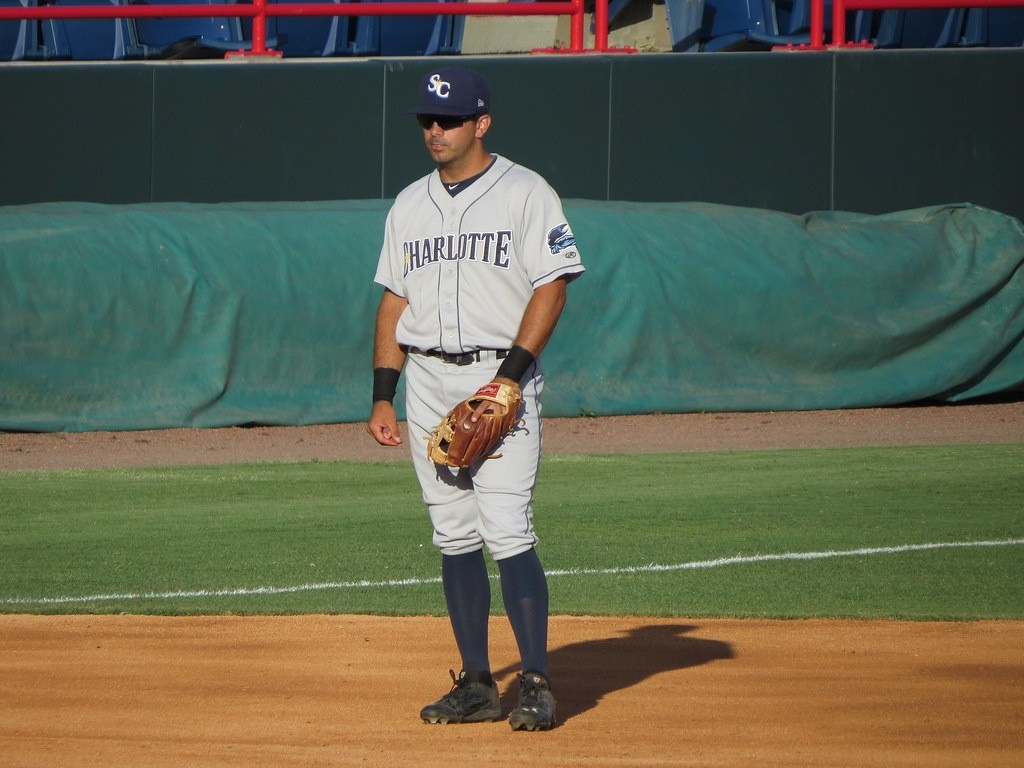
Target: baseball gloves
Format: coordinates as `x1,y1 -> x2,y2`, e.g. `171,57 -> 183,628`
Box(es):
424,375 -> 528,471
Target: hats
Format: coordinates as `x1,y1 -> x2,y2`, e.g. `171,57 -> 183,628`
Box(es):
403,66 -> 491,117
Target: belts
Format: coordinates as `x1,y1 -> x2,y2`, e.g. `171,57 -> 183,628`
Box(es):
411,346 -> 507,366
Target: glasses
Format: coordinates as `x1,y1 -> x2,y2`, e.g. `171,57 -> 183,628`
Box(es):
417,113 -> 478,130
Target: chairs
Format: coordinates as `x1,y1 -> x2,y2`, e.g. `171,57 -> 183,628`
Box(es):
1,0 -> 1024,55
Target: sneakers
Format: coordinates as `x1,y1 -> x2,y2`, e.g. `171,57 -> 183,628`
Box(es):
420,667 -> 502,725
508,669 -> 557,732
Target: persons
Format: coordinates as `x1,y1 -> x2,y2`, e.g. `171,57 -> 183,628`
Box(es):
366,68 -> 587,731
